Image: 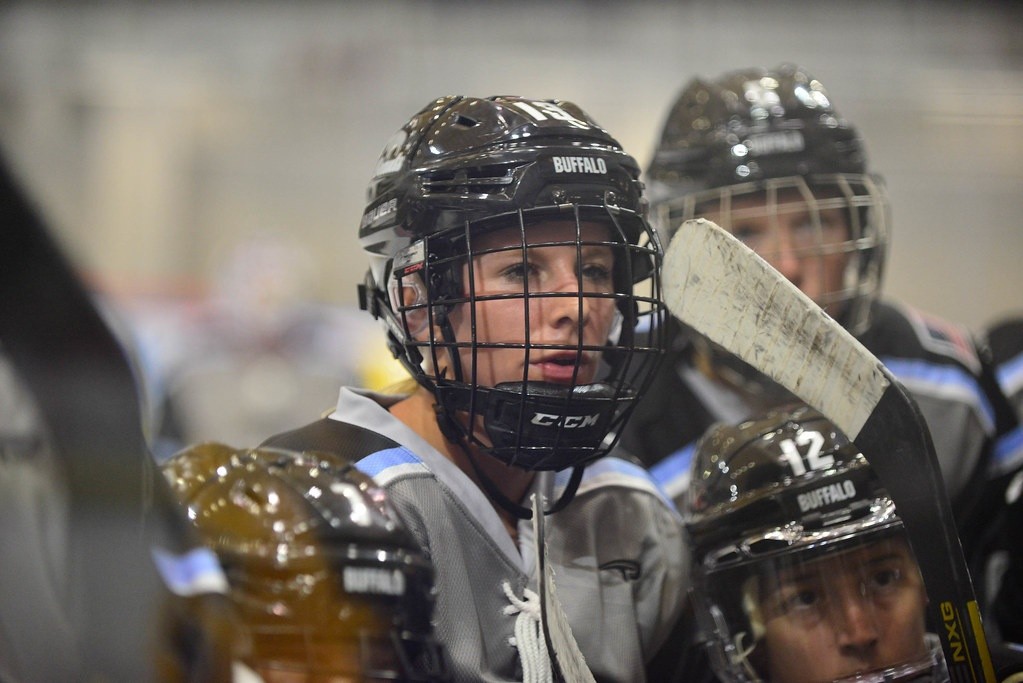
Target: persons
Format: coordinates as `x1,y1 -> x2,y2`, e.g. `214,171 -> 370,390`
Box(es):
159,444 -> 453,682
955,318 -> 1023,646
255,95 -> 690,683
607,64 -> 1017,502
0,156 -> 175,683
679,403 -> 952,683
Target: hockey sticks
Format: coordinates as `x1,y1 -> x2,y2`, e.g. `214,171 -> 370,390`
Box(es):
658,215 -> 1000,682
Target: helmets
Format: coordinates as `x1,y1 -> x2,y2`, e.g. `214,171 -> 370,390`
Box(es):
159,441 -> 457,683
643,64 -> 888,402
356,94 -> 669,473
681,403 -> 949,683
